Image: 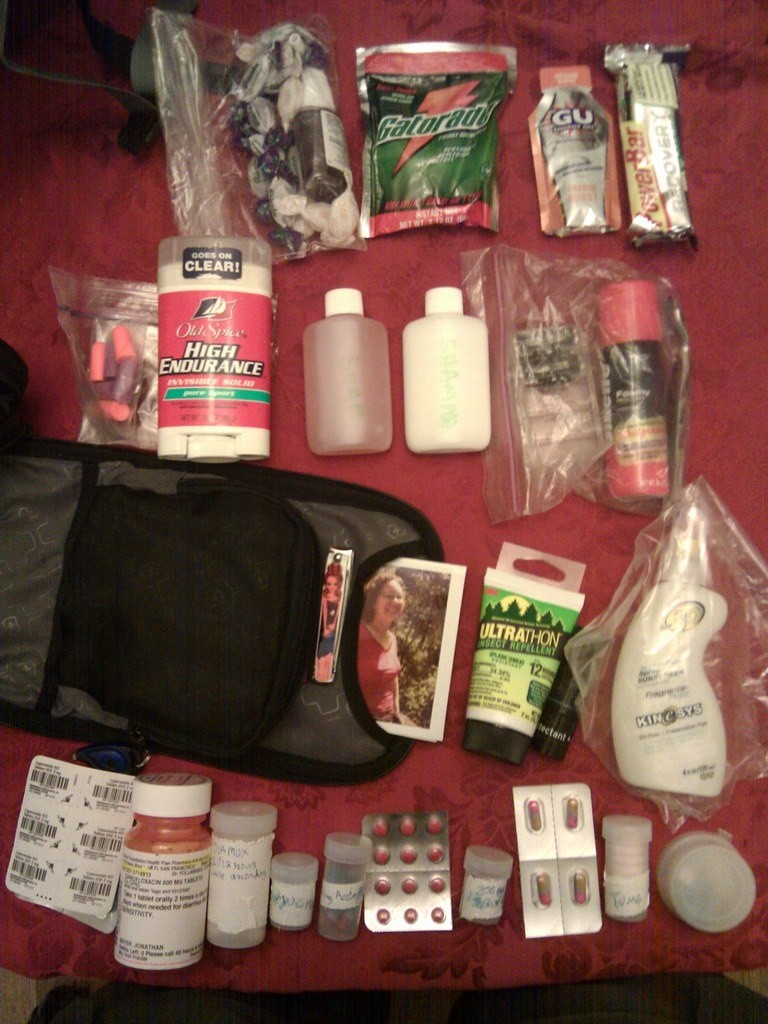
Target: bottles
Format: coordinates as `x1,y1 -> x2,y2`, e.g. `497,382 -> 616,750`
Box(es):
273,850 -> 320,930
401,284 -> 492,452
459,843 -> 514,925
114,770 -> 209,969
209,798 -> 277,949
319,830 -> 372,943
599,813 -> 651,922
303,285 -> 395,456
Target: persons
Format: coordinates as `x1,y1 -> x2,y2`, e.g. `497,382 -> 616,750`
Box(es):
357,571 -> 416,729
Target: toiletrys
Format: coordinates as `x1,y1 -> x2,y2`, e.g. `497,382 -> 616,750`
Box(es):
401,286 -> 491,455
610,505 -> 730,801
301,287 -> 393,458
461,566 -> 588,766
